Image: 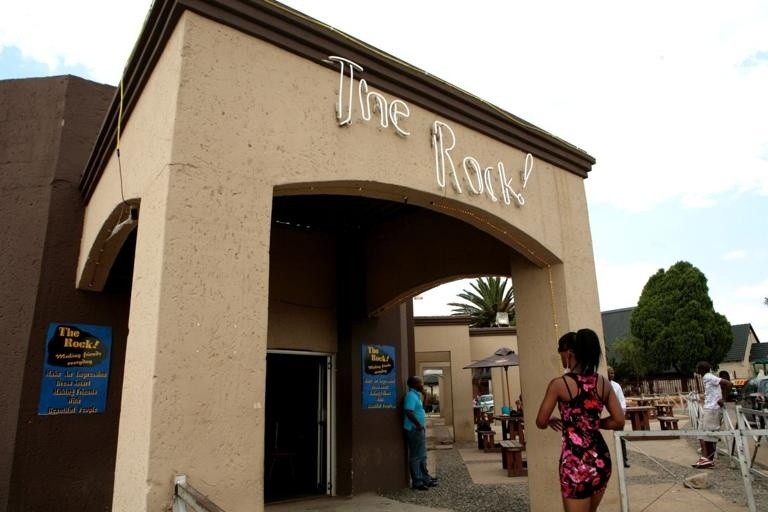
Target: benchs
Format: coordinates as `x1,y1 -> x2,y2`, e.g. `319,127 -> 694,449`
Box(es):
475,398 -> 680,477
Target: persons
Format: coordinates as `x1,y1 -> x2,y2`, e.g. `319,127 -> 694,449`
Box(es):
607,365 -> 631,468
692,362 -> 733,468
506,394 -> 523,432
404,375 -> 438,490
475,392 -> 484,406
536,329 -> 624,512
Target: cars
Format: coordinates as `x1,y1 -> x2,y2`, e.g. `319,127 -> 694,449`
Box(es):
478,395 -> 494,413
731,376 -> 768,441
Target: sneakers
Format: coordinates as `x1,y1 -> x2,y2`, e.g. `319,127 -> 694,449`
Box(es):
691,457 -> 714,468
413,476 -> 438,491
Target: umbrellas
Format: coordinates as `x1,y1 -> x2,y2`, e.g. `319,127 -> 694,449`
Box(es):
462,347 -> 521,411
